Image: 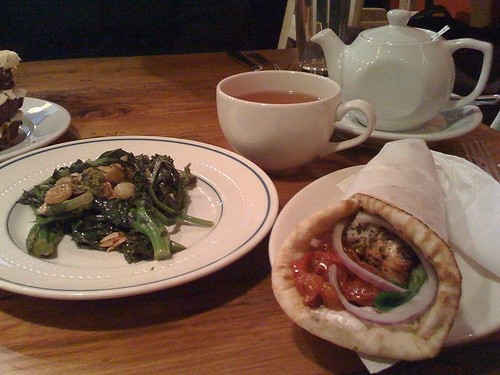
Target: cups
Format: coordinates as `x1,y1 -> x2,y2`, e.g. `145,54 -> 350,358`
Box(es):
216,70 -> 377,174
293,0 -> 352,77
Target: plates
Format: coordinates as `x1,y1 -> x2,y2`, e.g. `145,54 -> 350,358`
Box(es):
333,93 -> 483,144
266,164 -> 500,350
0,136 -> 280,301
0,97 -> 72,162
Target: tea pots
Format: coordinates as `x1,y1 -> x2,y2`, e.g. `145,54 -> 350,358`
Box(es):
309,9 -> 494,133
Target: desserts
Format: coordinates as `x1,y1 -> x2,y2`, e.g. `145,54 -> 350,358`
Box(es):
0,50 -> 26,152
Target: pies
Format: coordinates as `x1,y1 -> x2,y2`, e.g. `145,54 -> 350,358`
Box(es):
271,193 -> 464,361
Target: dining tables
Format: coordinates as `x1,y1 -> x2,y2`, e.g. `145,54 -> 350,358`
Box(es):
0,45 -> 500,375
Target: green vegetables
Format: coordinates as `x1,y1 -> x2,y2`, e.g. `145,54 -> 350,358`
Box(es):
15,147 -> 215,264
